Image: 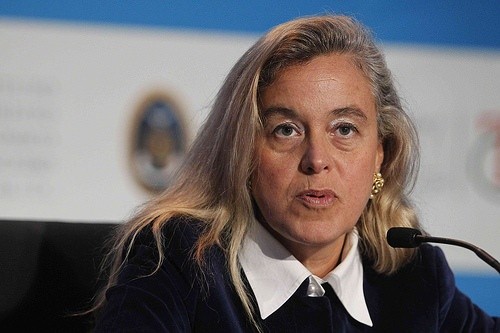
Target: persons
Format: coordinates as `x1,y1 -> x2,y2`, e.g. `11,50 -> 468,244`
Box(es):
95,14 -> 499,333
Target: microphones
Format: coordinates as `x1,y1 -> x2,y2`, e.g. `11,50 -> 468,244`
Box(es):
386,227 -> 500,275
